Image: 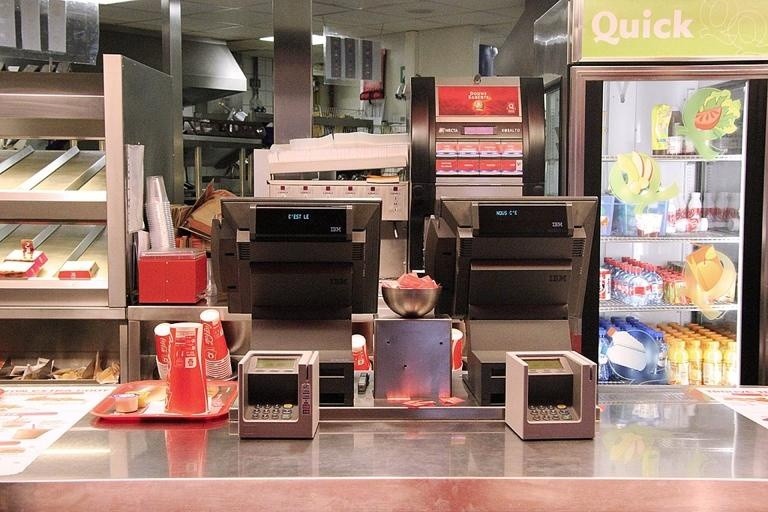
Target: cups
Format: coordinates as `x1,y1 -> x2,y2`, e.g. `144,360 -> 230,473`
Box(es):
451,328 -> 464,386
352,335 -> 369,372
152,308 -> 235,413
143,175 -> 176,249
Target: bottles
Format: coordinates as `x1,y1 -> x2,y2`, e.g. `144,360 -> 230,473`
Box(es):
601,316 -> 740,385
667,190 -> 711,235
706,191 -> 739,229
601,316 -> 736,385
599,255 -> 686,308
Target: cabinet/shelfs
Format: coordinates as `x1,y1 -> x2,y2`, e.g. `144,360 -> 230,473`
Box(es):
0,54 -> 173,384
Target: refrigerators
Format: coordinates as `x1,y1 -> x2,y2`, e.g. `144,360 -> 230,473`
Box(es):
532,0 -> 767,389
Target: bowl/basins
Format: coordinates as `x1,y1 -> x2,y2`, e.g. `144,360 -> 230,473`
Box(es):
381,285 -> 444,316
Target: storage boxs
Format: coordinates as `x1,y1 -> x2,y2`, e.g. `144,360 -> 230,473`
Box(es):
138,247 -> 207,304
